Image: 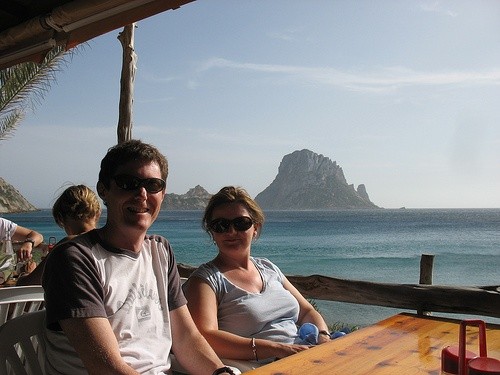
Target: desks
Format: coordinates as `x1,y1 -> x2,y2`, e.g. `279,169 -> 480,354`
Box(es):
239,310 -> 500,375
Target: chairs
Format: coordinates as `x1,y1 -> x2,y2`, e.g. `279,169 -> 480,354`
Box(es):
0,285 -> 49,375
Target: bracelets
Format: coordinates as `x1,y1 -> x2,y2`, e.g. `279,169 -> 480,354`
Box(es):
212,367 -> 235,375
23,238 -> 35,248
18,272 -> 31,277
252,337 -> 258,361
319,330 -> 331,339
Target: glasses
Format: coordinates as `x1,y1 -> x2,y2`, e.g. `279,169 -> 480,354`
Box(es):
206,216 -> 253,234
112,173 -> 165,194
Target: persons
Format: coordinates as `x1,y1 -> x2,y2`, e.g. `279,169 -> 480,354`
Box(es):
15,184 -> 102,286
0,218 -> 44,280
182,186 -> 332,365
41,140 -> 235,375
